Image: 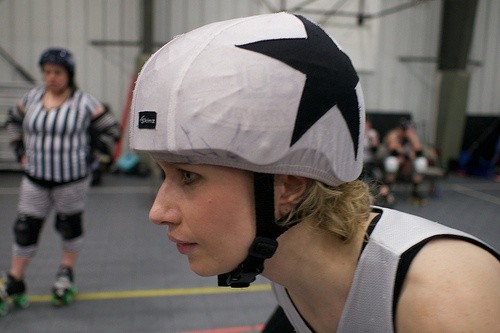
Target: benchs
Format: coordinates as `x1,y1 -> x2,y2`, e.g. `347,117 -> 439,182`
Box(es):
366,112 -> 443,198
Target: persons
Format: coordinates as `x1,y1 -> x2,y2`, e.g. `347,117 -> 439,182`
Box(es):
129,12 -> 500,333
377,118 -> 431,210
358,122 -> 389,204
0,48 -> 124,313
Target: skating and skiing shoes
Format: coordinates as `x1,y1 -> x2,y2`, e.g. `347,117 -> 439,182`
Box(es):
51,265 -> 77,305
0,273 -> 27,316
376,182 -> 396,206
409,183 -> 427,207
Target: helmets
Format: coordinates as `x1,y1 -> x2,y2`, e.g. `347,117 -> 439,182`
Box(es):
40,49 -> 74,77
130,12 -> 366,186
